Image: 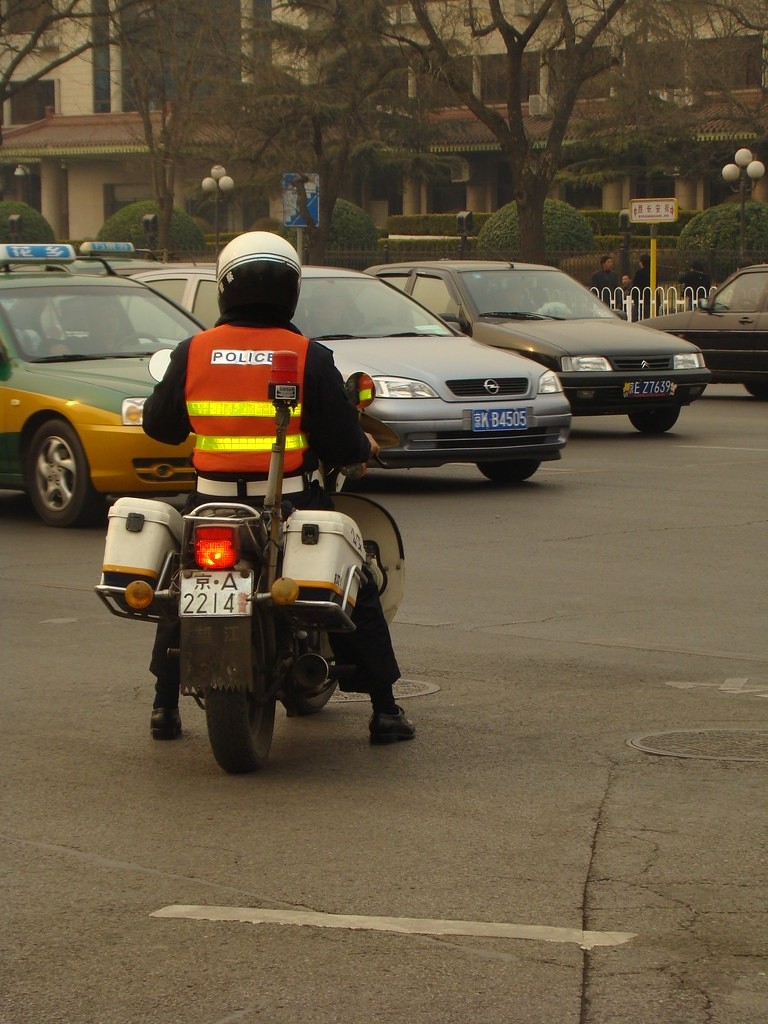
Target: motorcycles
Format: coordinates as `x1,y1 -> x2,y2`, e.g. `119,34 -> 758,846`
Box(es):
93,346 -> 405,778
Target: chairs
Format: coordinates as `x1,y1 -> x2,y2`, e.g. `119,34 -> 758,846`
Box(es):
58,297 -> 107,354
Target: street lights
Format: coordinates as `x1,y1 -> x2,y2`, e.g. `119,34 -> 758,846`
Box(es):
202,165 -> 232,254
720,148 -> 765,263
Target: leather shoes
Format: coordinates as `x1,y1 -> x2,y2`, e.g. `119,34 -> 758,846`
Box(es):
369,704 -> 416,745
150,709 -> 183,738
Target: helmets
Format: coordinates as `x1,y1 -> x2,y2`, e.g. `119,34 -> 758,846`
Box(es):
217,232 -> 302,323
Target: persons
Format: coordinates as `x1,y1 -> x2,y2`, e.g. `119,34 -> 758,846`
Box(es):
591,254 -> 768,323
37,294 -> 139,358
142,229 -> 418,746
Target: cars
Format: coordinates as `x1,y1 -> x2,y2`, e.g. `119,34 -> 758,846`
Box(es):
126,264 -> 574,487
631,263 -> 768,400
357,259 -> 711,436
0,242 -> 217,527
71,240 -> 164,281
